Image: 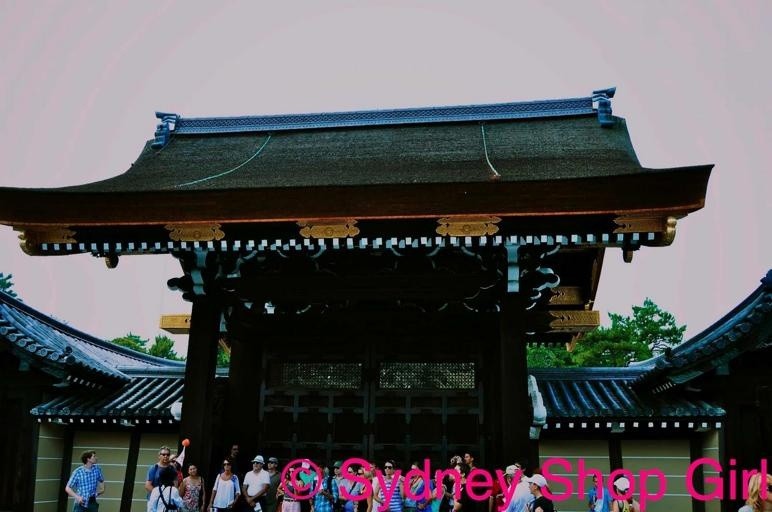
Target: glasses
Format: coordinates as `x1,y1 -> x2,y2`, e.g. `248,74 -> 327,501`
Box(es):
385,466 -> 392,469
253,462 -> 259,464
160,453 -> 170,456
223,463 -> 231,466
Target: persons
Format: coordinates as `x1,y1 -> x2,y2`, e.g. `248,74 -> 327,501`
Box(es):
609,476 -> 642,511
264,456 -> 283,511
147,467 -> 184,512
227,444 -> 247,479
277,450 -> 555,511
142,446 -> 175,492
177,463 -> 207,512
241,454 -> 271,511
170,453 -> 184,486
206,454 -> 242,512
64,449 -> 106,512
585,473 -> 615,511
739,471 -> 772,511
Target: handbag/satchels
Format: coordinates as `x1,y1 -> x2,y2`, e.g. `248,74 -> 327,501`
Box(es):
333,503 -> 346,512
162,504 -> 180,512
440,494 -> 451,512
198,489 -> 203,508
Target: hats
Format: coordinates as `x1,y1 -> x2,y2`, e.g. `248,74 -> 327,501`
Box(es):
614,477 -> 630,491
333,461 -> 342,468
251,455 -> 264,464
268,457 -> 278,463
301,459 -> 311,469
503,465 -> 519,476
527,474 -> 548,487
